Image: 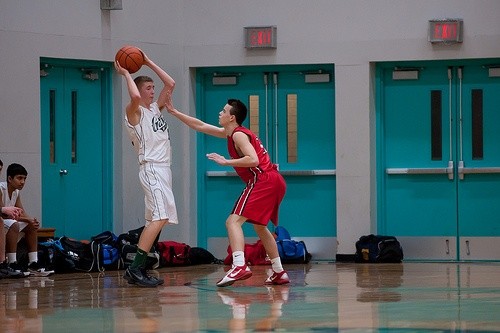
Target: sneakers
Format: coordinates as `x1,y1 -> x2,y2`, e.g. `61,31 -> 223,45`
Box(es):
216,263 -> 252,287
123,272 -> 164,287
263,270 -> 290,285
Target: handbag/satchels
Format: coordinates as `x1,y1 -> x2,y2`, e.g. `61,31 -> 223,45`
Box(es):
354,233 -> 404,262
36,226 -> 311,276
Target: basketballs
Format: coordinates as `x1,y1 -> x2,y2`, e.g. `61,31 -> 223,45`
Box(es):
116,46 -> 144,74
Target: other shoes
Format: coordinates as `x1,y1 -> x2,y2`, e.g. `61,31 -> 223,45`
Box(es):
8,262 -> 32,276
0,264 -> 23,278
29,262 -> 56,277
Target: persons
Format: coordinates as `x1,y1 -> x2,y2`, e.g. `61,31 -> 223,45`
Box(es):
114,47 -> 178,288
0,159 -> 24,279
164,89 -> 291,287
0,162 -> 55,277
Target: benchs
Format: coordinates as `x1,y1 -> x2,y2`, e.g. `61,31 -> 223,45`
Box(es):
36,228 -> 56,241
